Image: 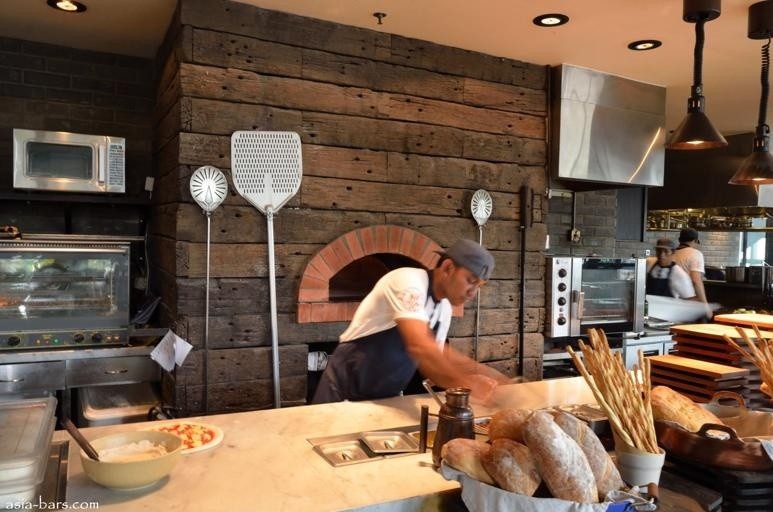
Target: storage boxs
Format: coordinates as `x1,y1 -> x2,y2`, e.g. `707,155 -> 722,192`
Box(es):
0,397 -> 57,509
79,384 -> 166,428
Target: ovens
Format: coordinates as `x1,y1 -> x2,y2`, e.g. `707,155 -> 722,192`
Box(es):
545,255 -> 646,340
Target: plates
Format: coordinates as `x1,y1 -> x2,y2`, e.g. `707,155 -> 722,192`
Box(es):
147,421 -> 224,454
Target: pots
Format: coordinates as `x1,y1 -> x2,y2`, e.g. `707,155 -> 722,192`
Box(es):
658,389 -> 773,471
706,265 -> 773,287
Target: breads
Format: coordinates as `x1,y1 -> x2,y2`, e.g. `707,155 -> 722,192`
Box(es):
651,385 -> 731,440
441,406 -> 626,506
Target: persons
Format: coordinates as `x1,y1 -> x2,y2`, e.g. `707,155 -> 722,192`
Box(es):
666,225 -> 714,321
309,236 -> 522,405
642,234 -> 699,302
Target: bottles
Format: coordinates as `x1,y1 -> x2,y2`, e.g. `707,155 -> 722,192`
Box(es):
421,385 -> 476,468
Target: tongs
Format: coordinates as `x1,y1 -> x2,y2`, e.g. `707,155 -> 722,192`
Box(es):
62,420 -> 100,463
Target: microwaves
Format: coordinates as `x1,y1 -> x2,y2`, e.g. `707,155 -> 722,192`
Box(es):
12,128 -> 127,194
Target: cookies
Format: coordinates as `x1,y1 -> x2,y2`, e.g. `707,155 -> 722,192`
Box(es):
723,322 -> 773,398
566,328 -> 660,456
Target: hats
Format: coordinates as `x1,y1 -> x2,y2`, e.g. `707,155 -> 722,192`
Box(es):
433,239 -> 495,280
679,228 -> 701,244
655,238 -> 676,250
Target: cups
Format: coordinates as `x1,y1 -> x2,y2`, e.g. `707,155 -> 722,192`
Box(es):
619,448 -> 666,495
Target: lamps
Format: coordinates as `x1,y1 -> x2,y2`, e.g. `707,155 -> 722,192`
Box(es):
663,0 -> 729,150
726,0 -> 773,184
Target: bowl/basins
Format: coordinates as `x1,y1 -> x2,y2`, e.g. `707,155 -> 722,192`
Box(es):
79,431 -> 182,492
